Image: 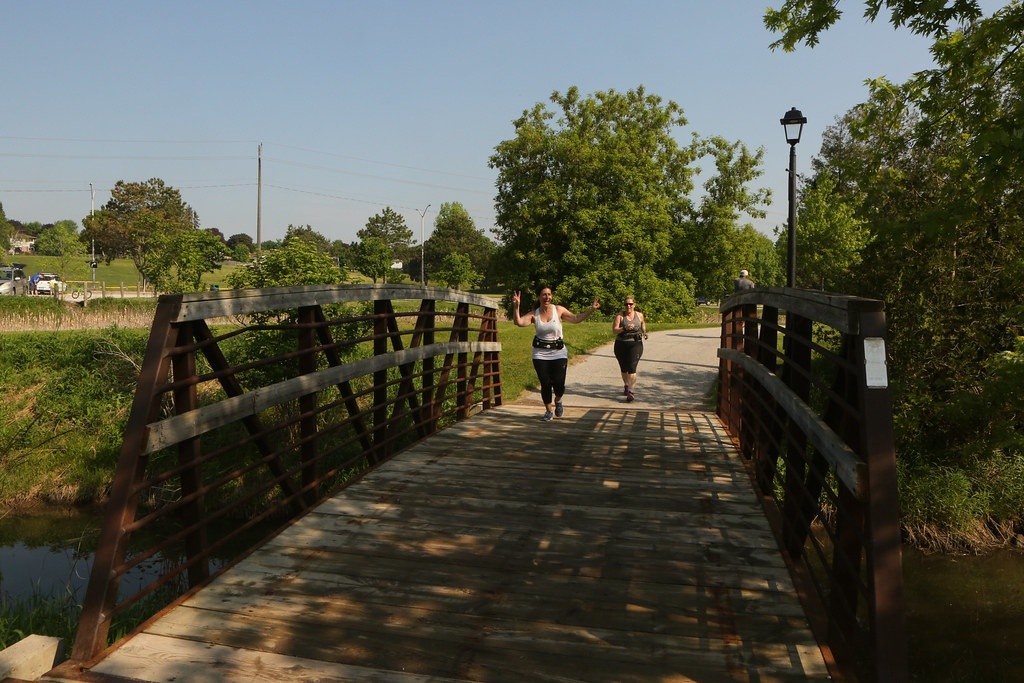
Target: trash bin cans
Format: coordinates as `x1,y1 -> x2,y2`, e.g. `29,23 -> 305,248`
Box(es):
210,284 -> 219,291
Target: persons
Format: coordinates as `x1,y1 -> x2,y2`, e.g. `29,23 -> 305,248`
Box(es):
513,285 -> 600,422
613,297 -> 648,402
735,270 -> 754,292
30,274 -> 44,297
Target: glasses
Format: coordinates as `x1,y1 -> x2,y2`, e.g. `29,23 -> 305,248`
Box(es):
624,302 -> 635,306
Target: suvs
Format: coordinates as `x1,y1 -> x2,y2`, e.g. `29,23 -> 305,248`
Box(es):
34,273 -> 67,296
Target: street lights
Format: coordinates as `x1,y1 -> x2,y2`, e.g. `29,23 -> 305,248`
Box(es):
779,105 -> 807,349
415,204 -> 432,289
89,182 -> 96,292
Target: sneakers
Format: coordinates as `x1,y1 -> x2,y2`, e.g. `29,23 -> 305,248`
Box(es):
624,385 -> 629,396
554,398 -> 563,417
540,411 -> 553,421
627,391 -> 635,401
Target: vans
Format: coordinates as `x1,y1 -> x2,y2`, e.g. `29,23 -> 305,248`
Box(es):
0,267 -> 28,295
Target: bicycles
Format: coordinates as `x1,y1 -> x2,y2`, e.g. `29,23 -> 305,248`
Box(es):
72,284 -> 92,300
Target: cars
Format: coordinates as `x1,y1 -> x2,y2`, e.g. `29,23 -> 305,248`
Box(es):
698,296 -> 711,305
